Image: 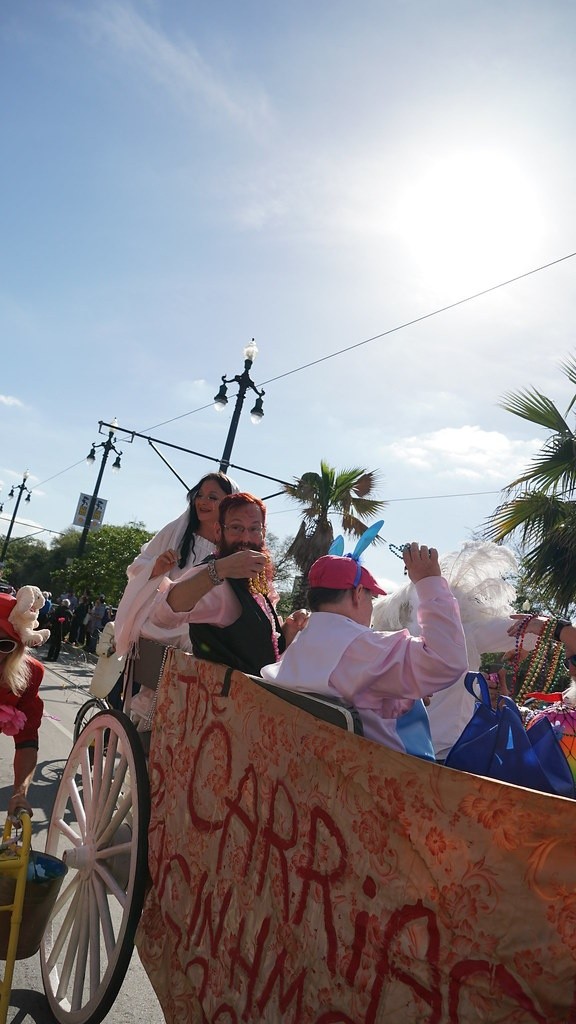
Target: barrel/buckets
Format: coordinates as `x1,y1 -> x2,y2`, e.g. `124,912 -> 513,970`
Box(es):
0,842 -> 70,961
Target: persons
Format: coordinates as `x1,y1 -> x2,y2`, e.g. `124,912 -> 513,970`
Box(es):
113,473 -> 240,659
482,614 -> 576,721
42,599 -> 73,662
149,492 -> 311,679
264,520 -> 469,762
0,593 -> 44,829
4,586 -> 20,597
39,591 -> 51,616
61,590 -> 78,610
67,590 -> 112,655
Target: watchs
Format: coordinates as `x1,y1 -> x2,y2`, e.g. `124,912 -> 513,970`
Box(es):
554,619 -> 571,642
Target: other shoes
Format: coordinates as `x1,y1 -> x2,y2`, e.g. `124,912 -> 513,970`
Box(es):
42,657 -> 58,662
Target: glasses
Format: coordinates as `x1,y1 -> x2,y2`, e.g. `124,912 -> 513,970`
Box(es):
222,523 -> 266,538
563,655 -> 576,669
0,639 -> 18,654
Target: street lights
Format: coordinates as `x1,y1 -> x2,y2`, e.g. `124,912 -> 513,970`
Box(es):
212,337 -> 267,475
67,415 -> 124,596
0,468 -> 33,563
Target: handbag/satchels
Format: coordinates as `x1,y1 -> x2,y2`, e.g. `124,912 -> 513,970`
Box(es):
444,671 -> 575,800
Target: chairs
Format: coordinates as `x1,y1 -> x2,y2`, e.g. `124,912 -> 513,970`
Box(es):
245,673 -> 364,737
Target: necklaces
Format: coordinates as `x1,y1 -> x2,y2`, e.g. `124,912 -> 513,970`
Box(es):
248,554 -> 270,594
251,593 -> 279,663
509,614 -> 566,709
387,540 -> 408,574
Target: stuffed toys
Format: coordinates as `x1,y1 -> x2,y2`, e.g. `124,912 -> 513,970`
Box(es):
8,585 -> 50,646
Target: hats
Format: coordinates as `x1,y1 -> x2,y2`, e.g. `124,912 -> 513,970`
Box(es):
0,585 -> 22,641
308,555 -> 388,598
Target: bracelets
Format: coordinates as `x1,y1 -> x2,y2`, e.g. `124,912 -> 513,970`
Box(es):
208,559 -> 224,586
